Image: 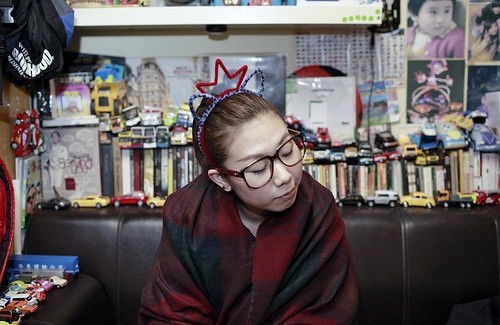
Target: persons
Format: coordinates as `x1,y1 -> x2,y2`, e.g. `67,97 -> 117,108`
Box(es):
138,88 -> 359,325
468,66 -> 499,114
412,60 -> 453,104
468,0 -> 500,61
46,131 -> 67,200
408,0 -> 464,58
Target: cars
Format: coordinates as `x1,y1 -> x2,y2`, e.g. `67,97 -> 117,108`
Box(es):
36,197 -> 70,210
0,276 -> 80,325
70,195 -> 110,209
146,196 -> 165,206
286,110 -> 500,165
111,192 -> 148,208
335,194 -> 366,207
400,191 -> 436,209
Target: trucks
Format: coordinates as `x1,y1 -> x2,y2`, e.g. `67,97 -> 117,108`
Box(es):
436,190 -> 500,209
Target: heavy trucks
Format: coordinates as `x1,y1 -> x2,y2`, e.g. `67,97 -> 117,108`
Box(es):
20,269 -> 74,281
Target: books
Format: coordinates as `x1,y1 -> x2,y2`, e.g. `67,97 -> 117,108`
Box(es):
10,153 -> 43,256
40,126 -> 102,204
100,137 -> 203,201
301,147 -> 499,201
42,115 -> 99,128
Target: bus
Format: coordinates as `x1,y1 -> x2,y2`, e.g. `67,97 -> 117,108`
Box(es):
90,64 -> 193,148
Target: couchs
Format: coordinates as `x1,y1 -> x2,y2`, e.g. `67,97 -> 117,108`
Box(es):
18,205 -> 500,325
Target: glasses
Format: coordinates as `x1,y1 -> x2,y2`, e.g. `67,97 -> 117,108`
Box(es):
217,127 -> 307,189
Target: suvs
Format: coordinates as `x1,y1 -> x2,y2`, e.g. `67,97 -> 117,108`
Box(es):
365,190 -> 400,208
